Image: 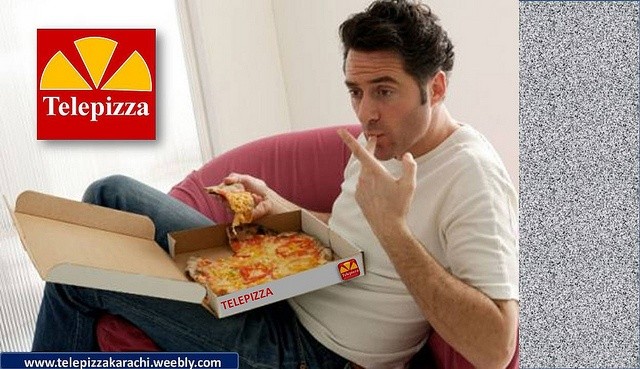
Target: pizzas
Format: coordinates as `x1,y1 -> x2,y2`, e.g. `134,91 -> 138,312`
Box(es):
186,224 -> 335,296
202,183 -> 255,236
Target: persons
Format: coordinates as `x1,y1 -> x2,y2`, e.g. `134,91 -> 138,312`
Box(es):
32,1 -> 519,369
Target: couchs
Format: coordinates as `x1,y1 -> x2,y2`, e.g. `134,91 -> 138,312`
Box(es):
94,125 -> 518,368
